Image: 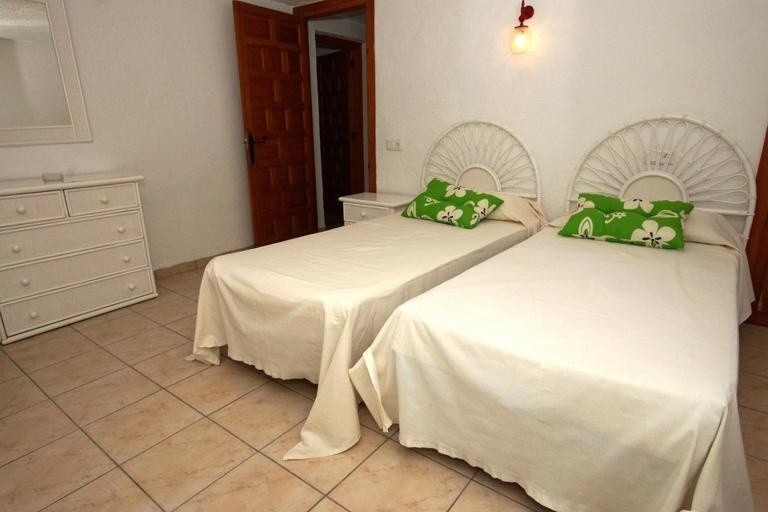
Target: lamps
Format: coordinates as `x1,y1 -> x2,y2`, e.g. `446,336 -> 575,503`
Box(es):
510,0 -> 534,54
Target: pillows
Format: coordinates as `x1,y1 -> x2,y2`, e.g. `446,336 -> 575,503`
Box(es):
401,179 -> 503,229
548,208 -> 742,252
476,189 -> 547,237
556,193 -> 693,249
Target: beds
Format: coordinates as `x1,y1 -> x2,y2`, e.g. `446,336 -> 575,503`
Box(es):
187,122 -> 543,460
347,116 -> 754,512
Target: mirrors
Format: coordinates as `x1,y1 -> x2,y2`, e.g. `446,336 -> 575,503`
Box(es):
0,0 -> 93,146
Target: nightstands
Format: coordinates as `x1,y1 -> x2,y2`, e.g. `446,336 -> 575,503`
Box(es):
338,192 -> 415,227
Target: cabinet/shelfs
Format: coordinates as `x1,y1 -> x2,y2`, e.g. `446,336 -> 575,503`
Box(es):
0,172 -> 159,346
